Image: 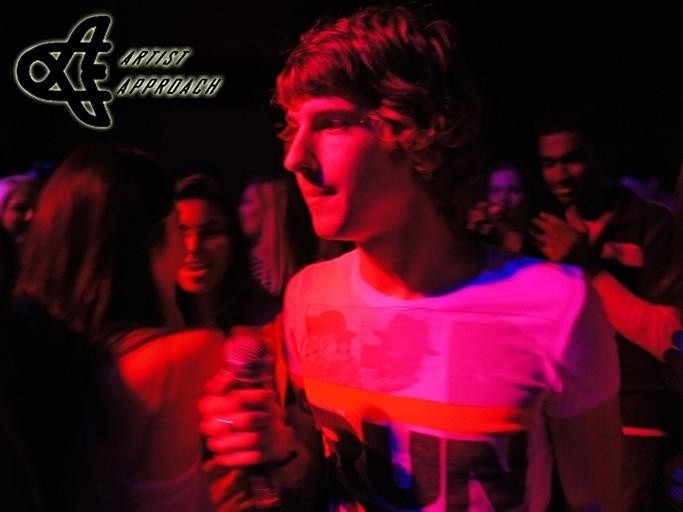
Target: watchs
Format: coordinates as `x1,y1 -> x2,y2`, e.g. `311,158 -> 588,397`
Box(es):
274,425 -> 300,468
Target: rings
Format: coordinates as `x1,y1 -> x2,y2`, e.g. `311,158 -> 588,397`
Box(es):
216,412 -> 233,432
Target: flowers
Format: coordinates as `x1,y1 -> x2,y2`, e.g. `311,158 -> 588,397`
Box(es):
223,336 -> 276,496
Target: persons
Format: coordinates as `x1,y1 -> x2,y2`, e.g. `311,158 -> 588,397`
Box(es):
2,169 -> 38,232
2,302 -> 88,510
467,163 -> 530,255
196,6 -> 626,512
530,103 -> 682,368
14,151 -> 233,512
239,180 -> 298,295
174,173 -> 281,334
